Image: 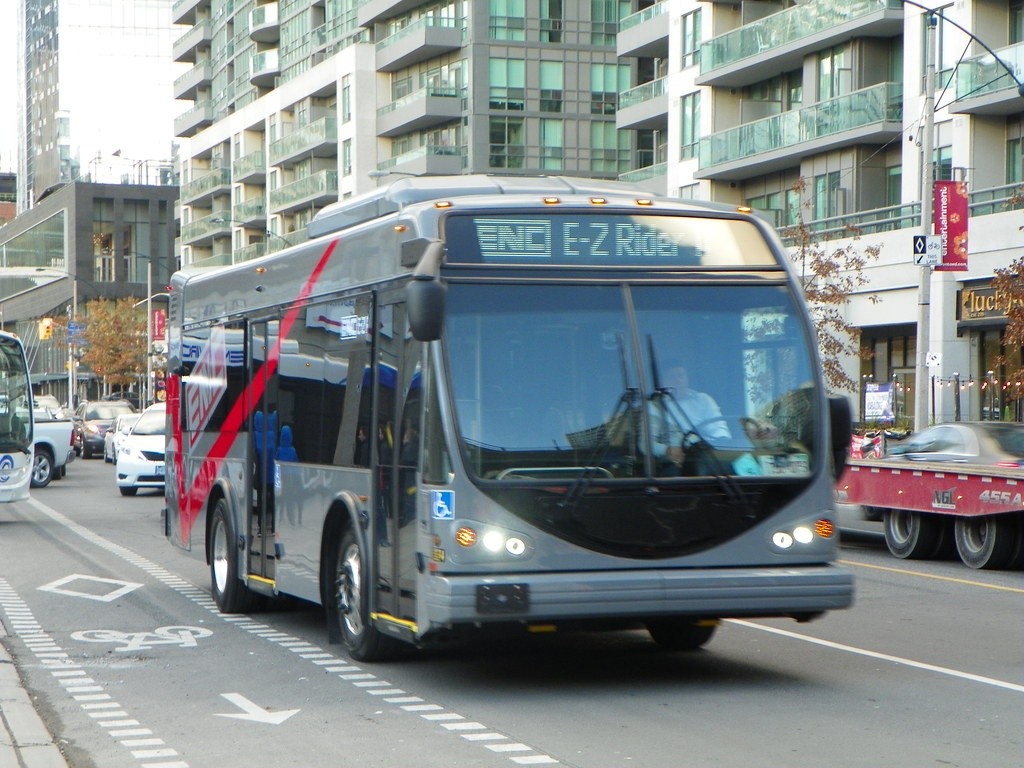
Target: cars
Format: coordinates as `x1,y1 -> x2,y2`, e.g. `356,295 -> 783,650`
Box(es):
104,413 -> 142,465
0,393 -> 64,422
116,402 -> 165,496
886,420 -> 1024,469
103,392 -> 138,408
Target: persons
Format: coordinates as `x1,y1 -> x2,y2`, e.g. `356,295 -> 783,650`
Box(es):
639,359 -> 731,479
357,424 -> 417,548
253,410 -> 299,536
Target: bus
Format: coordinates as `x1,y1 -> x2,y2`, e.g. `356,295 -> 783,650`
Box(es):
0,330 -> 34,502
161,173 -> 860,666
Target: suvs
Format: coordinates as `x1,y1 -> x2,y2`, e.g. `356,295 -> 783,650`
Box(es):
71,399 -> 137,459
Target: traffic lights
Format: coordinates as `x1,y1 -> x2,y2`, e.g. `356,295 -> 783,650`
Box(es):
43,317 -> 53,336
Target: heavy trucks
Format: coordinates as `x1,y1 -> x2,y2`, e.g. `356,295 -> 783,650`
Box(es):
831,457 -> 1024,569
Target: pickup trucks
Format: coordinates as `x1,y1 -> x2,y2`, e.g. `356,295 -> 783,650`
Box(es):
18,417 -> 75,488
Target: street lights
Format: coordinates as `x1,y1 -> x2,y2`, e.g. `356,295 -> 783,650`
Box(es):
36,268 -> 107,396
914,9 -> 1024,432
209,219 -> 293,249
